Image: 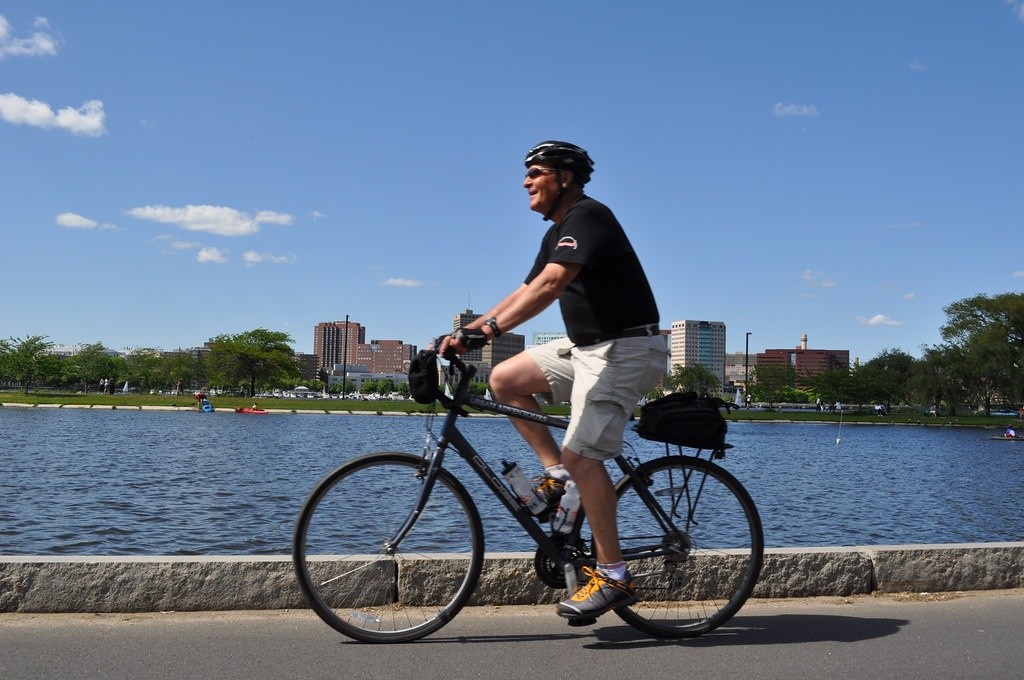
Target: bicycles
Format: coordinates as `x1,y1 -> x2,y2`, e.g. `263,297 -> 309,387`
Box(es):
290,334 -> 765,644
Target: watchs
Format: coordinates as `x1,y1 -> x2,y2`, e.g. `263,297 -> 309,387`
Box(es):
483,316 -> 502,339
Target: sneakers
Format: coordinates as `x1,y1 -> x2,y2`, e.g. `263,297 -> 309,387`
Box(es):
529,471 -> 565,523
557,565 -> 640,615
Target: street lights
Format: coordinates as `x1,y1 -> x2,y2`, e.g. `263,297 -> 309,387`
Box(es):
342,315 -> 351,400
745,333 -> 753,408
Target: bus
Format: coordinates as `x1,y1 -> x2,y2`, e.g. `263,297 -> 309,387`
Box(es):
293,386 -> 309,392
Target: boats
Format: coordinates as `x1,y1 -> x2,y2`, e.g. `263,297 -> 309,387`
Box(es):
236,407 -> 269,414
991,435 -> 1024,441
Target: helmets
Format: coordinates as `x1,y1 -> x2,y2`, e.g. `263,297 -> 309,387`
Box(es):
525,140 -> 595,183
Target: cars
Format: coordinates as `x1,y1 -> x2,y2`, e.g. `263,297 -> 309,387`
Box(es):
149,388 -> 416,402
990,409 -> 1018,416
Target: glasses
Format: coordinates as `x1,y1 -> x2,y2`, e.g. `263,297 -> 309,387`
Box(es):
524,167 -> 566,178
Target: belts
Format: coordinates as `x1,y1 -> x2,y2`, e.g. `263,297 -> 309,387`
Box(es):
579,324 -> 660,348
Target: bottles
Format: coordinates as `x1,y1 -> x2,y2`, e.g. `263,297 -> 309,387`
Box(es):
554,481 -> 581,533
501,459 -> 548,514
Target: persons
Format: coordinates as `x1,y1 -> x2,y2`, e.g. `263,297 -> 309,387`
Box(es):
252,403 -> 259,411
98,376 -> 248,399
744,393 -> 938,419
1005,425 -> 1022,438
438,141 -> 667,621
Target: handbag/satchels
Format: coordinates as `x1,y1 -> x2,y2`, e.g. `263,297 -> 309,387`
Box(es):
636,390 -> 727,449
